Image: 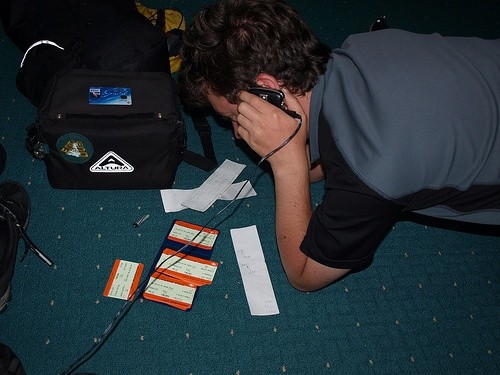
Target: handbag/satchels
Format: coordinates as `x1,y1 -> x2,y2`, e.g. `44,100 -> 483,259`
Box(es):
26,67 -> 218,190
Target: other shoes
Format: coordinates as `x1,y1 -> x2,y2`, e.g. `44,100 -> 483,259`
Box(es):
0,180 -> 30,236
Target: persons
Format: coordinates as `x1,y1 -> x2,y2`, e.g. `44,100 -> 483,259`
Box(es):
180,1 -> 500,292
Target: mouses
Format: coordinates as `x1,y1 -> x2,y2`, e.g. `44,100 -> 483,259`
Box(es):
166,28 -> 186,56
